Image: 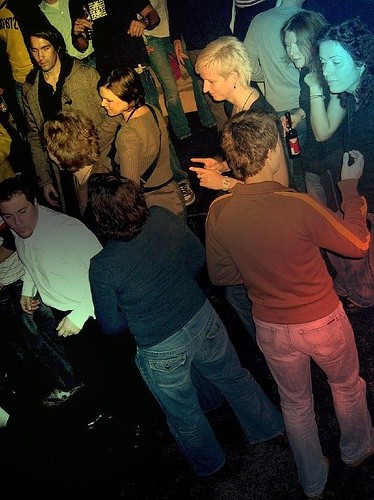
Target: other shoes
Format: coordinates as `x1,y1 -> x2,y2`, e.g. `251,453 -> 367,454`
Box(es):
41,386 -> 82,408
177,178 -> 196,206
342,443 -> 374,469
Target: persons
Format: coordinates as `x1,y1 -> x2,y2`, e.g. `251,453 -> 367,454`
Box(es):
0,0 -> 374,336
87,173 -> 285,477
1,175 -> 103,409
206,111 -> 374,500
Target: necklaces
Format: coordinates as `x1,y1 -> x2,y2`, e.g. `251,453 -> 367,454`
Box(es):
233,88 -> 254,118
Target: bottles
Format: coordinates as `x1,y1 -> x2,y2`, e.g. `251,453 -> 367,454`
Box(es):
83,6 -> 95,41
284,111 -> 301,159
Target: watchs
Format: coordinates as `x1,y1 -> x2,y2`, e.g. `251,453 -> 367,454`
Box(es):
223,175 -> 230,191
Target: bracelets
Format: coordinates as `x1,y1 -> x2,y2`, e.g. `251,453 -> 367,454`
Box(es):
143,17 -> 151,28
310,94 -> 323,99
